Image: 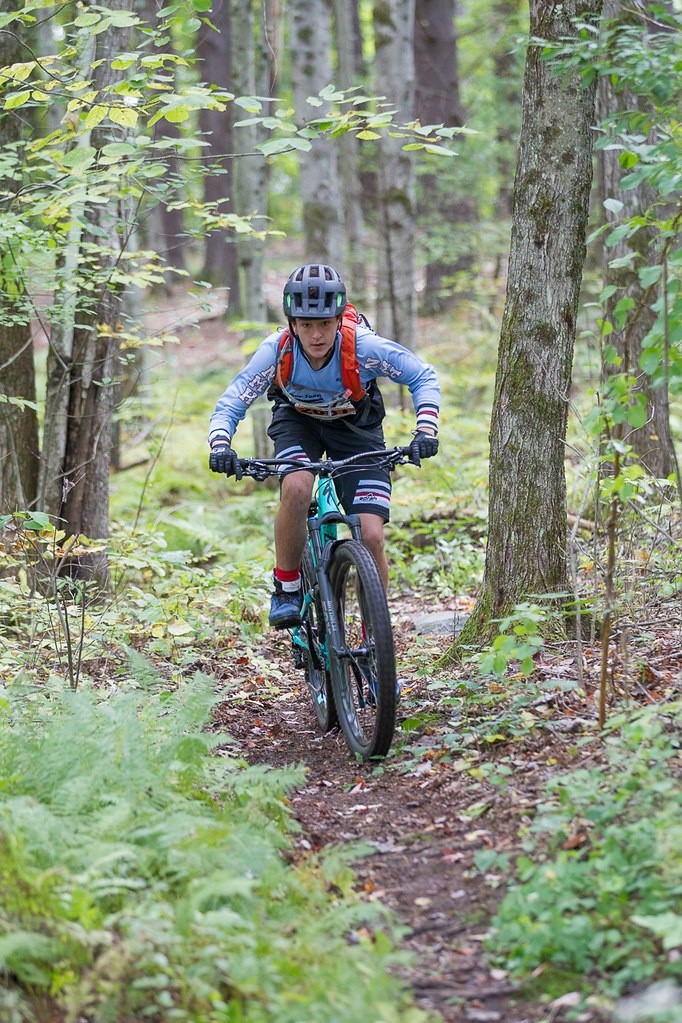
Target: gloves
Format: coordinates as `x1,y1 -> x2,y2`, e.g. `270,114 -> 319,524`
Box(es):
209,444 -> 242,480
408,429 -> 438,464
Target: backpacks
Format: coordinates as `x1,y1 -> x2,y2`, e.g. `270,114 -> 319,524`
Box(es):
266,304 -> 372,422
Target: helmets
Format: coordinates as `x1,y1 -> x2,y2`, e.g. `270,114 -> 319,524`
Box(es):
283,264 -> 346,317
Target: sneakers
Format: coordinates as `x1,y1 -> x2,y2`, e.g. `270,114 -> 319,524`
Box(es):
358,647 -> 400,708
269,590 -> 301,625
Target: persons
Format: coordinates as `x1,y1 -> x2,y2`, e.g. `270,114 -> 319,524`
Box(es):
208,264 -> 441,709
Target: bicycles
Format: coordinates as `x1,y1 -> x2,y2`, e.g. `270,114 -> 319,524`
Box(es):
232,444 -> 424,766
